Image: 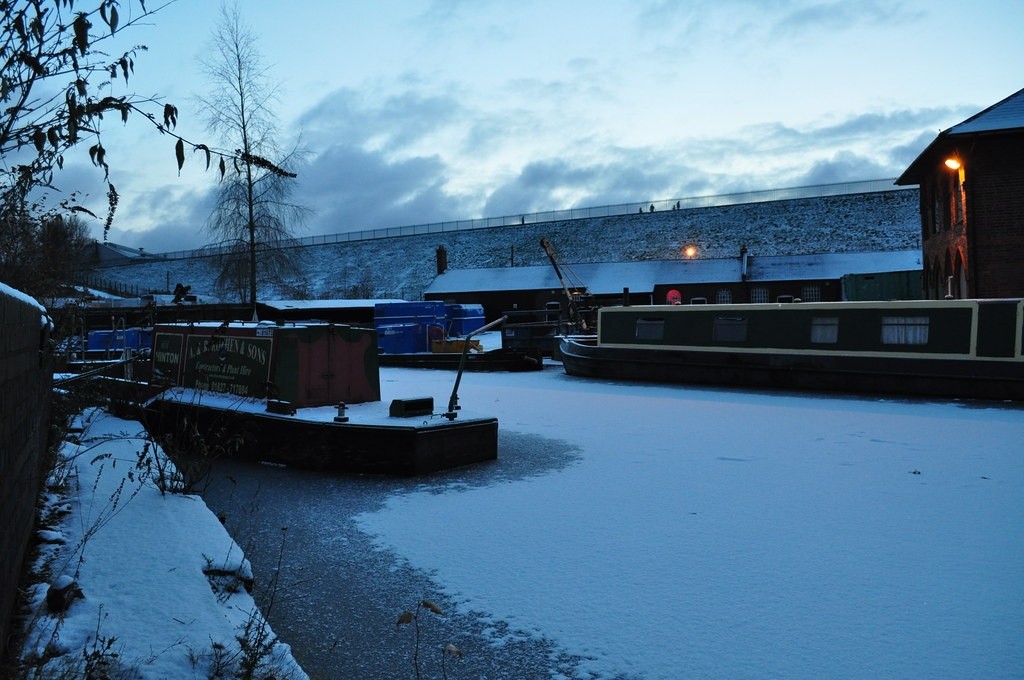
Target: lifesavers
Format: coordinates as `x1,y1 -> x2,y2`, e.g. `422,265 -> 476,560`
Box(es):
428,326 -> 445,344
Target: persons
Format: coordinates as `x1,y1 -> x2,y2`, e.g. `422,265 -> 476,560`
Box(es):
672,200 -> 680,210
649,204 -> 655,212
639,207 -> 642,213
522,216 -> 524,224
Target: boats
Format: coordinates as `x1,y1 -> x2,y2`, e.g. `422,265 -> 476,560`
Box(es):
66,131 -> 545,479
560,295 -> 1024,398
